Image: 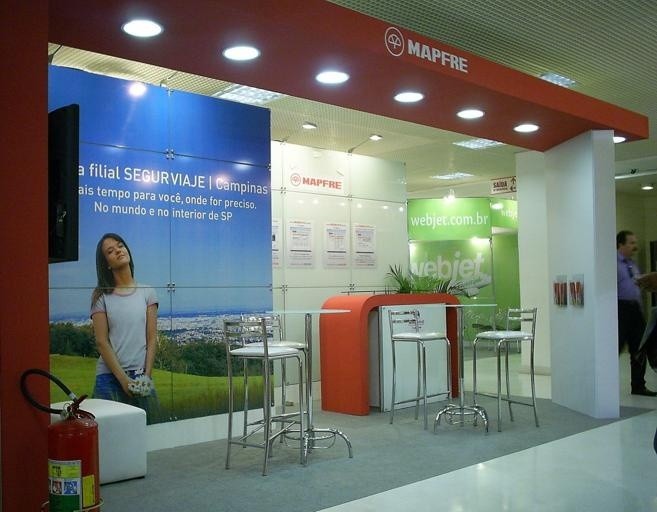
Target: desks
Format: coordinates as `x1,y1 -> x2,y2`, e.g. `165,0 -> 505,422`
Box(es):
434,304 -> 497,433
265,309 -> 353,464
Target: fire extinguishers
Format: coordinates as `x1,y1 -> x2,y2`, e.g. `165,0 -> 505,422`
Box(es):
20,368 -> 105,511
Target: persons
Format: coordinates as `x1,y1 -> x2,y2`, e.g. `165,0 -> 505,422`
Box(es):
615,230 -> 655,398
89,232 -> 160,426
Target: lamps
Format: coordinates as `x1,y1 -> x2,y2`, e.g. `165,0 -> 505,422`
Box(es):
274,121 -> 317,142
347,133 -> 384,153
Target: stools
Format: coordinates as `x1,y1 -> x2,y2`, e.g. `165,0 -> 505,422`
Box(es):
50,398 -> 147,485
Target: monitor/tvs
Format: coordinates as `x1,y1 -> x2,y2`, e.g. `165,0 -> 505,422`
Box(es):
48,103 -> 80,264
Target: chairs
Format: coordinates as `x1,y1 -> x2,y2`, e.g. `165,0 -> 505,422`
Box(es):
223,319 -> 304,476
388,310 -> 452,430
240,314 -> 306,448
473,308 -> 540,432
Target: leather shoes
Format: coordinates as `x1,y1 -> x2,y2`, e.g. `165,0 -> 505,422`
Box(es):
632,386 -> 657,396
